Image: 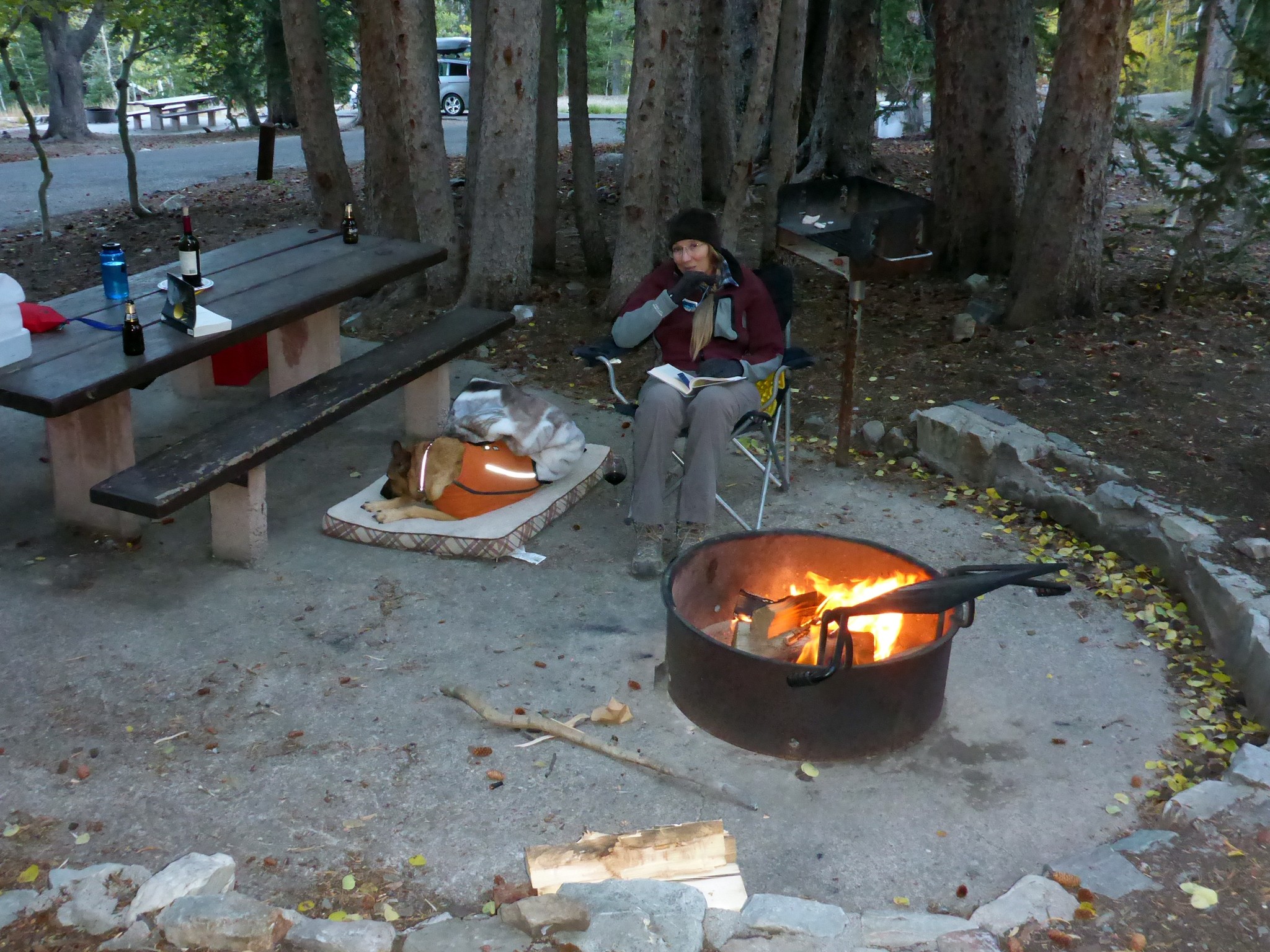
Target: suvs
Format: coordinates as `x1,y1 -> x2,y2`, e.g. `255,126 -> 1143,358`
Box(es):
349,37 -> 471,115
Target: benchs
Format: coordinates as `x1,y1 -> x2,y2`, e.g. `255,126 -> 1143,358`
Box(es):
90,304 -> 516,569
126,106 -> 187,129
157,106 -> 228,131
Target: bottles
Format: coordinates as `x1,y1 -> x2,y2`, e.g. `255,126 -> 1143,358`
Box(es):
341,202 -> 358,244
178,206 -> 203,286
121,299 -> 146,355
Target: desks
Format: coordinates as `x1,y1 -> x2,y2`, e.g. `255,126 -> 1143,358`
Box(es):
1,224 -> 450,541
127,94 -> 218,130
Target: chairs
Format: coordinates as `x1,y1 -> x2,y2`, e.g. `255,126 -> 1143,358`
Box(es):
570,266 -> 814,533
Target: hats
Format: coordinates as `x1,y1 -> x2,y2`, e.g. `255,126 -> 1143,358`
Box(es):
668,208 -> 723,255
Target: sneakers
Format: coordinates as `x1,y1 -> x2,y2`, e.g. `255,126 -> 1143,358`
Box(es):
672,518 -> 708,562
629,520 -> 667,581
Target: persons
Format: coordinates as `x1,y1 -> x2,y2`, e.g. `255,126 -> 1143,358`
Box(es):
607,210 -> 789,577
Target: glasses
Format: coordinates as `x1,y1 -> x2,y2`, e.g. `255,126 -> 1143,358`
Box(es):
667,240 -> 707,258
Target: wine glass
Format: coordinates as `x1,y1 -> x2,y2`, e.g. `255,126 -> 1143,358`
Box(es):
602,455 -> 627,507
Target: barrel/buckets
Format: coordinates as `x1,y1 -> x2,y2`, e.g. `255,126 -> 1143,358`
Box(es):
657,527 -> 974,757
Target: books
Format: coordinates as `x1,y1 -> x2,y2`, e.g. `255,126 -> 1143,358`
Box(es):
160,272 -> 233,337
645,363 -> 748,398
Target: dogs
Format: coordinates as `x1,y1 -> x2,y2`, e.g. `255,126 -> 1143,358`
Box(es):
361,440 -> 589,523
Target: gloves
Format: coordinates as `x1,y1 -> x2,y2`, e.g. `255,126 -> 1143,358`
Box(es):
668,270 -> 716,305
697,359 -> 744,378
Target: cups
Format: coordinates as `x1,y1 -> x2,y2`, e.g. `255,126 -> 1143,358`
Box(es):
99,240 -> 129,298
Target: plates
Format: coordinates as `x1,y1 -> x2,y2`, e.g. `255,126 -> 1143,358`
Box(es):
158,276 -> 214,291
